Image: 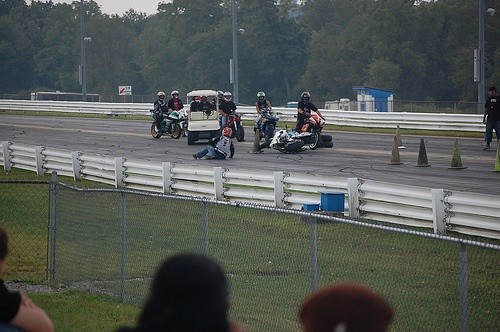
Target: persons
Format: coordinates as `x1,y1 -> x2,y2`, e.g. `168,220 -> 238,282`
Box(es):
483,87 -> 500,150
190,91 -> 237,124
193,127 -> 234,160
256,92 -> 272,129
295,92 -> 325,133
118,252 -> 237,332
299,280 -> 396,332
168,91 -> 183,112
154,92 -> 168,131
0,227 -> 55,332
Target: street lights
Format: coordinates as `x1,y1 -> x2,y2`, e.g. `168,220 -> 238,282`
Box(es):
477,8 -> 496,114
80,36 -> 92,102
232,28 -> 245,105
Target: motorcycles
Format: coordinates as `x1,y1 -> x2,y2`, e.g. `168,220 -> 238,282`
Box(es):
187,89 -> 220,145
253,106 -> 326,154
150,111 -> 189,139
218,111 -> 247,142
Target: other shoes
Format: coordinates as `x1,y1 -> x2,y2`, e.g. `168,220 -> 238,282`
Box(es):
193,154 -> 197,159
483,144 -> 491,149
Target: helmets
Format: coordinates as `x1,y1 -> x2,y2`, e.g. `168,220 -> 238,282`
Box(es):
217,91 -> 223,99
257,92 -> 266,102
171,91 -> 179,98
223,92 -> 232,101
222,127 -> 232,137
301,92 -> 311,102
158,91 -> 166,98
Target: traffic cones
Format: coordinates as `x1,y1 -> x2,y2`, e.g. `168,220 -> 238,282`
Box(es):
414,138 -> 431,167
396,124 -> 407,149
447,138 -> 469,169
388,135 -> 404,165
490,147 -> 500,172
